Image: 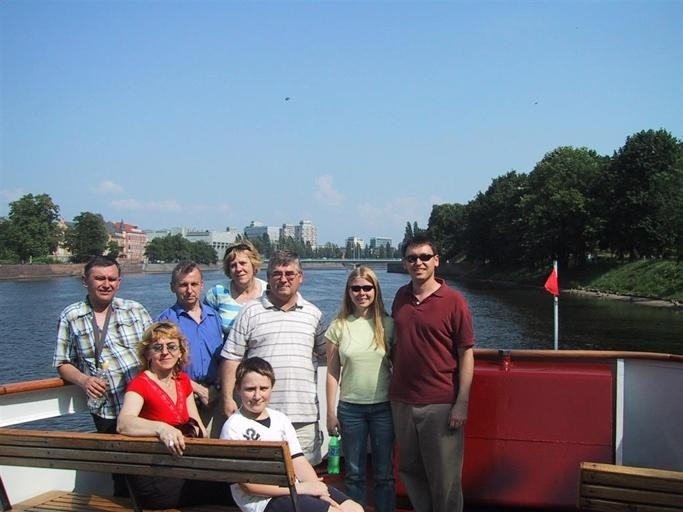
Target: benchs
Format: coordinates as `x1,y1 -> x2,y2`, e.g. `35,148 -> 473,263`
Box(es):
575,458 -> 683,512
0,428 -> 300,512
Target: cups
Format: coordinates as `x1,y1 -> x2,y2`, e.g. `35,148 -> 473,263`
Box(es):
497,347 -> 510,360
501,360 -> 511,372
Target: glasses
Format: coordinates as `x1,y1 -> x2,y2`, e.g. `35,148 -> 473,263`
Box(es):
406,255 -> 436,262
350,286 -> 375,292
223,244 -> 253,259
269,272 -> 300,280
152,343 -> 180,351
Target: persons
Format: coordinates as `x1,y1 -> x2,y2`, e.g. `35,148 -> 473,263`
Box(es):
51,257 -> 156,498
113,320 -> 227,509
220,356 -> 365,512
153,262 -> 225,440
202,238 -> 270,340
220,252 -> 327,469
324,265 -> 397,511
391,235 -> 475,512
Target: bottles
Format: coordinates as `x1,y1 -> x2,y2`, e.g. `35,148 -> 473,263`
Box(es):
327,430 -> 342,476
87,362 -> 109,409
